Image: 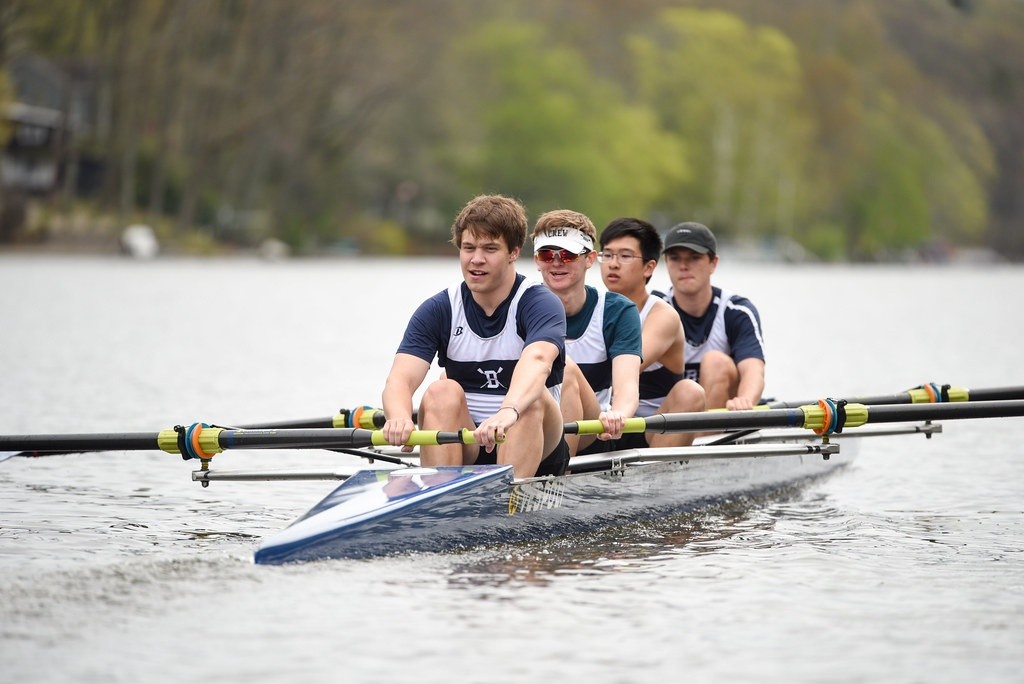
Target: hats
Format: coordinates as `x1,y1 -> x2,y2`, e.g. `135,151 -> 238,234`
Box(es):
661,222 -> 716,255
533,226 -> 594,256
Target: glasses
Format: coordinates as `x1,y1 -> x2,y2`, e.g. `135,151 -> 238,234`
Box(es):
536,248 -> 589,263
597,251 -> 643,264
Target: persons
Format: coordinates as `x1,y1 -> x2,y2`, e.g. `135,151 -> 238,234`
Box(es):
600,217 -> 706,446
382,196 -> 569,480
530,209 -> 645,458
664,222 -> 766,434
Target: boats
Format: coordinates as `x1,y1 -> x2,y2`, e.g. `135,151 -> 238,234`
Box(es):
252,414 -> 856,573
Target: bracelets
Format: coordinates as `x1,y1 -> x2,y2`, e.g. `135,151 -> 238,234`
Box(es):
498,407 -> 519,421
